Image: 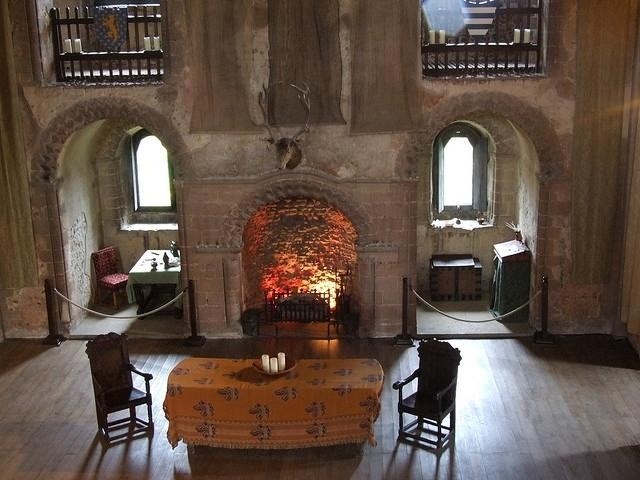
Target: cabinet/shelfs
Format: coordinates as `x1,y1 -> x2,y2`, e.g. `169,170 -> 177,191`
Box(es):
489,240 -> 532,322
429,254 -> 482,300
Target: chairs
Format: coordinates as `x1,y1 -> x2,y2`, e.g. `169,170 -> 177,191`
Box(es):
392,337 -> 463,456
90,246 -> 128,312
84,332 -> 155,449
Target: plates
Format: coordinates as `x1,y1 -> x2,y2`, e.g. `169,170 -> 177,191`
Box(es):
253,354 -> 296,375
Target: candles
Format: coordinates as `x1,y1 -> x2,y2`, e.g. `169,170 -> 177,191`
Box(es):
524,29 -> 530,44
65,39 -> 72,53
439,29 -> 446,44
143,37 -> 152,51
262,354 -> 269,371
429,29 -> 436,45
278,352 -> 286,371
270,358 -> 278,372
514,28 -> 520,44
153,36 -> 161,50
74,39 -> 82,54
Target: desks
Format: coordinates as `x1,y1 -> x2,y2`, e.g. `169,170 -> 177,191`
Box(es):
162,358 -> 384,452
128,248 -> 182,320
